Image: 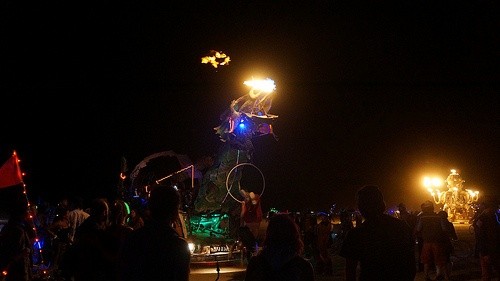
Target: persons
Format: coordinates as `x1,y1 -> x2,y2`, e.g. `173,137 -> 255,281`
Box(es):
228,198 -> 500,281
120,186 -> 191,281
0,196 -> 133,281
339,185 -> 417,281
240,187 -> 263,238
